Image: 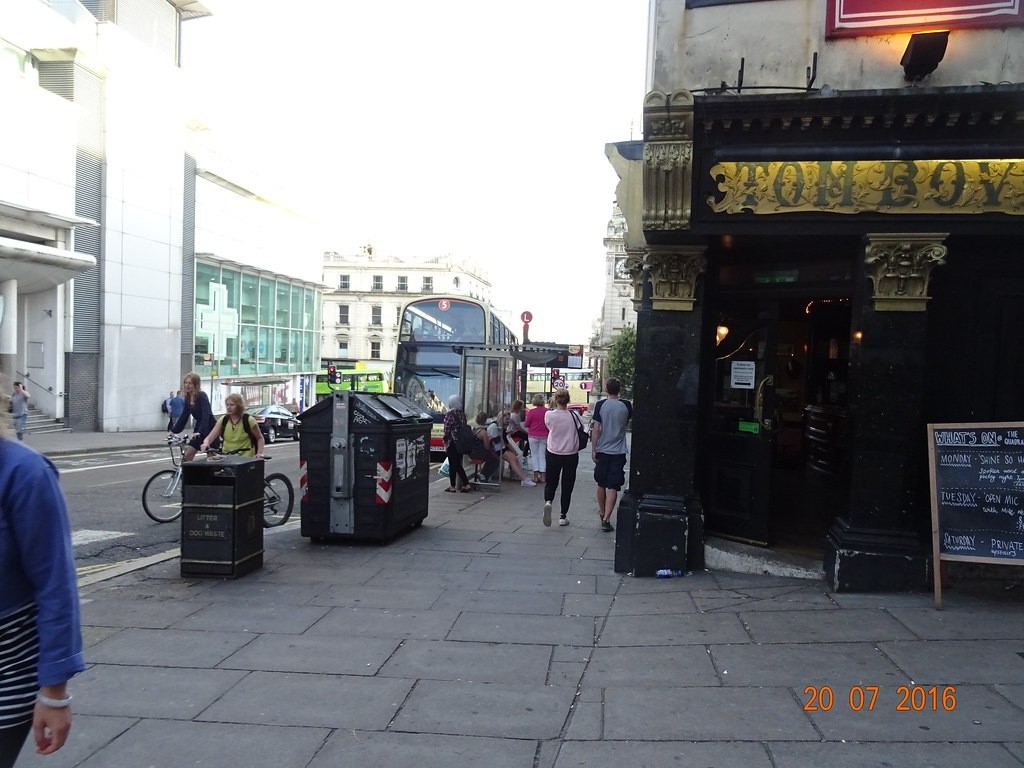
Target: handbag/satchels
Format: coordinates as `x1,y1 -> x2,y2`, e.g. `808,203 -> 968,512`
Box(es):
438,457 -> 450,478
8,391 -> 15,413
570,409 -> 589,451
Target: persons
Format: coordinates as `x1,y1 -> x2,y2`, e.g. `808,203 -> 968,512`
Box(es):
442,394 -> 472,493
200,392 -> 265,458
543,388 -> 584,527
165,391 -> 175,432
487,409 -> 537,487
548,397 -> 558,411
0,437 -> 87,768
409,328 -> 425,342
166,372 -> 222,477
169,390 -> 185,426
524,393 -> 551,484
426,327 -> 439,341
467,412 -> 500,482
507,399 -> 530,464
591,377 -> 633,532
10,382 -> 30,440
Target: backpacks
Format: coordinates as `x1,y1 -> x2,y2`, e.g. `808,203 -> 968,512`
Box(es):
451,410 -> 472,453
162,399 -> 173,412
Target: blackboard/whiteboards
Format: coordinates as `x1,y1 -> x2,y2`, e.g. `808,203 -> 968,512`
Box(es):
927,422 -> 1024,565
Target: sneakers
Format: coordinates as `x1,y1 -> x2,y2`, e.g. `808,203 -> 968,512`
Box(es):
521,478 -> 537,487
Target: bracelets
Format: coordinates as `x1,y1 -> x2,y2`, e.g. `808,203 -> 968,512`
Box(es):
36,690 -> 73,708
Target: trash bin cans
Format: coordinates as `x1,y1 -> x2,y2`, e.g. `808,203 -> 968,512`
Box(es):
295,389 -> 433,547
181,454 -> 265,580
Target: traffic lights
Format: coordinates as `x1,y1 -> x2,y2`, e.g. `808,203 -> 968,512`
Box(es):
329,365 -> 336,384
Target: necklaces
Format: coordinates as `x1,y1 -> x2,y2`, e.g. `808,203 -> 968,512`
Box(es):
229,416 -> 241,431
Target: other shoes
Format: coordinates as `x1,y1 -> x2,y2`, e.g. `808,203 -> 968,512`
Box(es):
559,518 -> 569,526
533,475 -> 544,483
476,473 -> 489,484
543,503 -> 552,526
599,510 -> 613,531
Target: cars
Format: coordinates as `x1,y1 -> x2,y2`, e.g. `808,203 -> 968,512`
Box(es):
244,405 -> 303,443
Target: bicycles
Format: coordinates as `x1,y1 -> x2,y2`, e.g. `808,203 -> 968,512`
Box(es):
142,434 -> 294,527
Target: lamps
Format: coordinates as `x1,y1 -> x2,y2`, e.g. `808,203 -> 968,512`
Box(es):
407,327 -> 482,340
715,312 -> 729,345
901,30 -> 951,81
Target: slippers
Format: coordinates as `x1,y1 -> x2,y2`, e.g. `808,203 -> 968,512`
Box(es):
460,486 -> 468,492
445,487 -> 457,493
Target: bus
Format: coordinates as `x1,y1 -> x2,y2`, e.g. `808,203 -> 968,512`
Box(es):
526,368 -> 602,412
387,293 -> 522,453
315,368 -> 393,404
